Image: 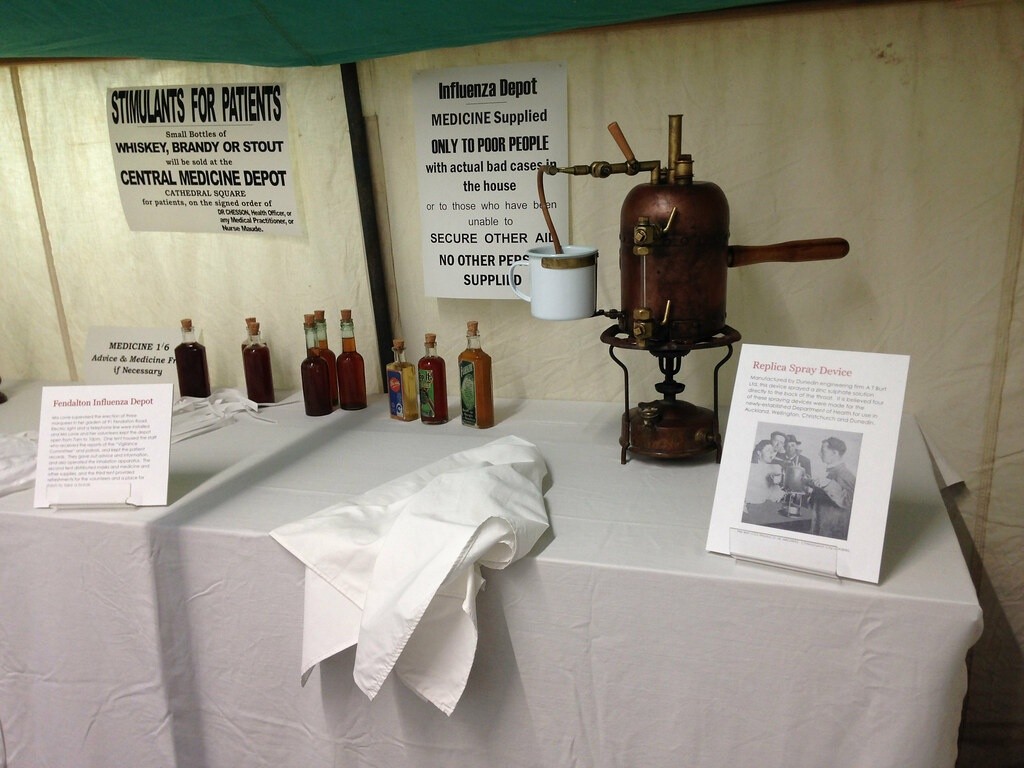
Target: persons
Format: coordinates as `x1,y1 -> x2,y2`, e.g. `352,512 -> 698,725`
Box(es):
742,431 -> 857,541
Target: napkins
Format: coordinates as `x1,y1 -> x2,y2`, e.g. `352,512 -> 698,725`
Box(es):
268,436 -> 551,718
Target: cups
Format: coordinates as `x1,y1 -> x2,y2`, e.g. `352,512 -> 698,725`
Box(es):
508,245 -> 599,321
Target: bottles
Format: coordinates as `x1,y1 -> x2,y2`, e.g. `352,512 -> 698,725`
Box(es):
315,310 -> 338,411
242,322 -> 275,407
386,337 -> 420,422
175,319 -> 211,398
300,314 -> 333,416
417,333 -> 448,425
458,321 -> 494,429
337,309 -> 367,410
241,318 -> 267,350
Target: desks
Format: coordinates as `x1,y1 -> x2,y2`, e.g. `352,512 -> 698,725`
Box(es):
0,393 -> 984,768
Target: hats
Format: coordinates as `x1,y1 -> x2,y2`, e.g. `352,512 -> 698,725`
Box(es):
784,435 -> 801,448
